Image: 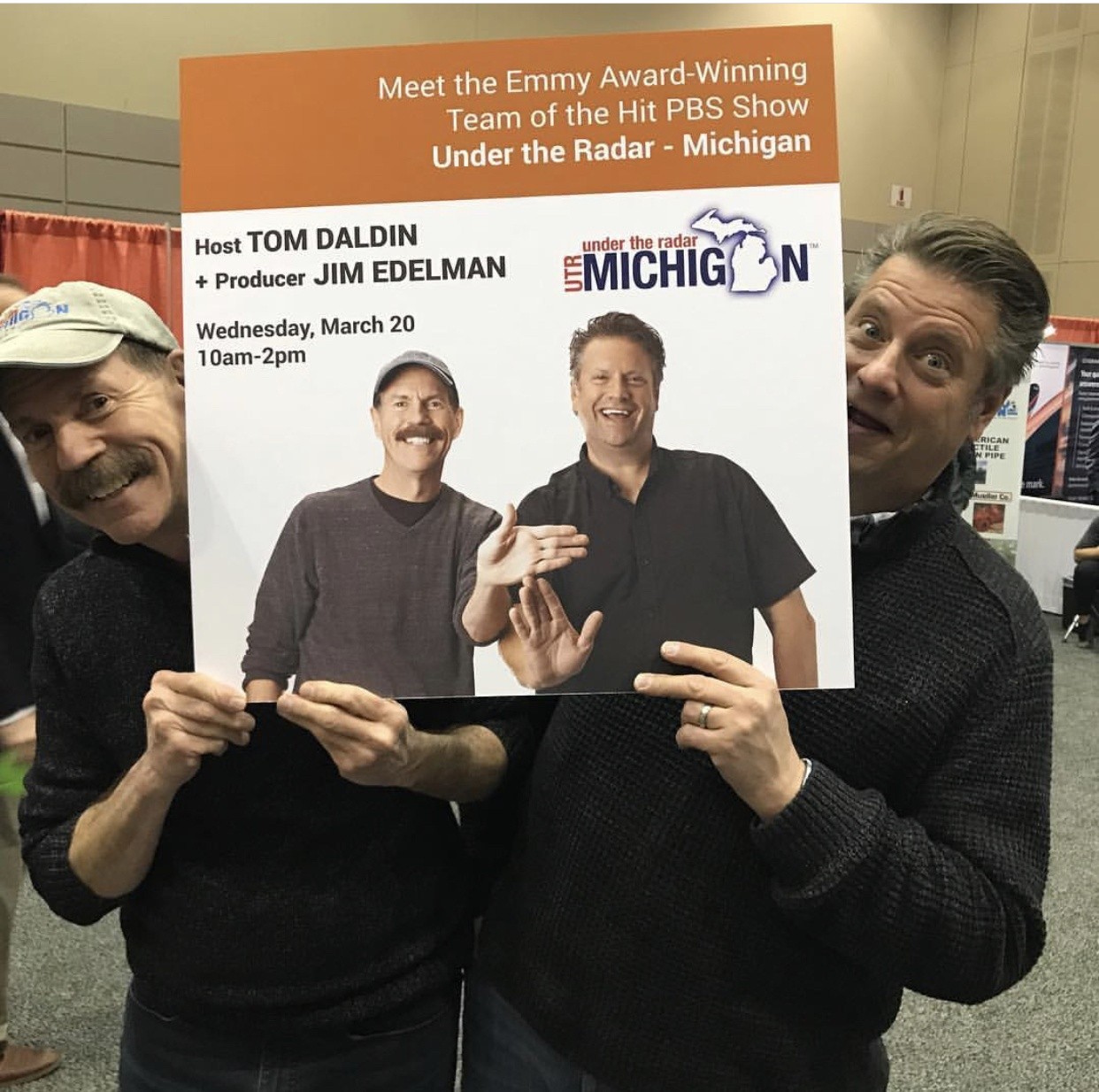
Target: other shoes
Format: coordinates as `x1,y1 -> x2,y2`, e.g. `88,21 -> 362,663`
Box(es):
1079,614 -> 1090,625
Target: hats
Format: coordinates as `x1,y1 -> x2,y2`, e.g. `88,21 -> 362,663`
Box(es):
376,351 -> 454,385
0,281 -> 182,366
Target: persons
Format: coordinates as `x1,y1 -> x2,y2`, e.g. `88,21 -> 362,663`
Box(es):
1073,512 -> 1099,649
5,278 -> 563,1088
0,272 -> 107,1087
493,303 -> 817,695
236,348 -> 587,702
462,212 -> 1057,1083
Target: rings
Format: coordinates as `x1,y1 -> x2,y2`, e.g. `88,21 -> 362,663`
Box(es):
702,701 -> 714,733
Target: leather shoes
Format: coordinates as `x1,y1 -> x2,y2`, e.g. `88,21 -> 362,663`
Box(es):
0,1047 -> 64,1085
